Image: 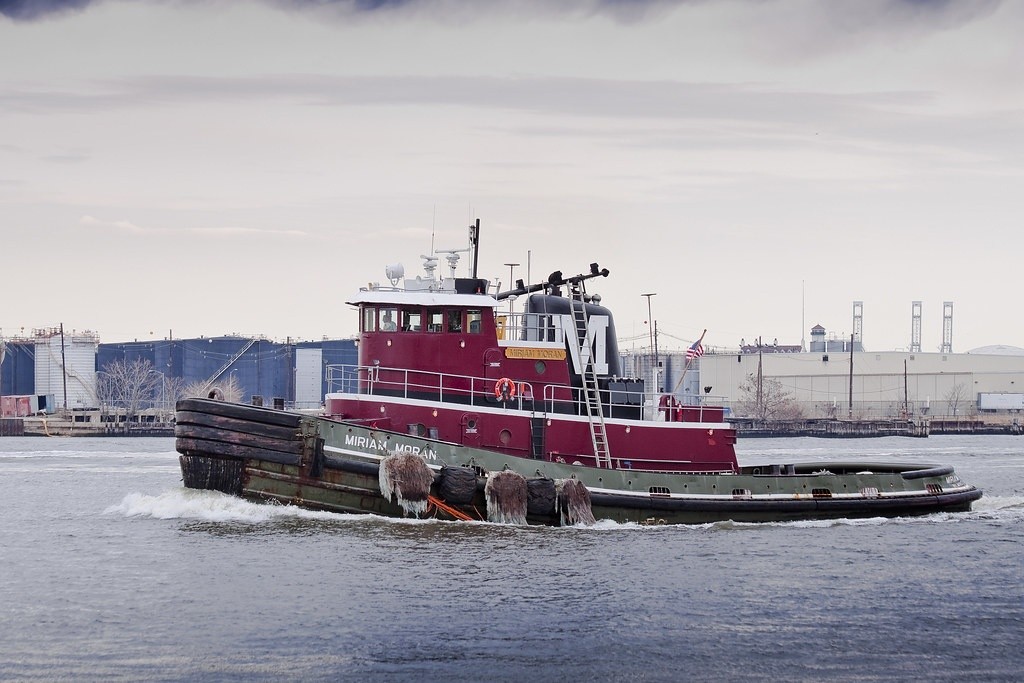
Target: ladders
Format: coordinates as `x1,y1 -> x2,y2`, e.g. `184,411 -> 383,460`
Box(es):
566,279 -> 613,469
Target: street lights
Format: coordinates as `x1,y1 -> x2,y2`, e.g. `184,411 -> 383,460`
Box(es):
503,263 -> 520,339
641,293 -> 657,367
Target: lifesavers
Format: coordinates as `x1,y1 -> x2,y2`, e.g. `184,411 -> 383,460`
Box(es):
494,377 -> 516,402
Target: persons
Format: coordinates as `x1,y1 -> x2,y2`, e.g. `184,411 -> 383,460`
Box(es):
382,315 -> 397,330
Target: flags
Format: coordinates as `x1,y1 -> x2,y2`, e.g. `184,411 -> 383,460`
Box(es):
685,340 -> 703,359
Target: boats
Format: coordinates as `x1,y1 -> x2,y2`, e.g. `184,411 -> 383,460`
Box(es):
173,201 -> 985,527
